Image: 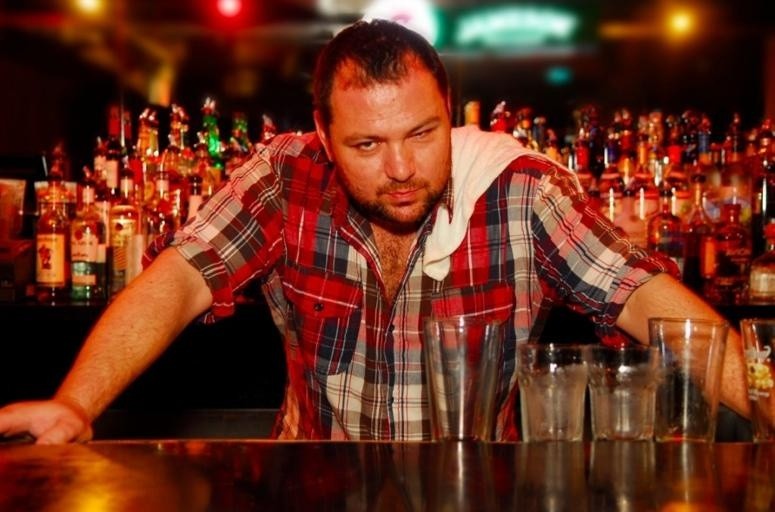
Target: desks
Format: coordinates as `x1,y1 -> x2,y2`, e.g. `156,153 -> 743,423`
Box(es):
0,433 -> 774,511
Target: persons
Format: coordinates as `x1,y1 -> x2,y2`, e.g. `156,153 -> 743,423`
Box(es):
0,21 -> 775,445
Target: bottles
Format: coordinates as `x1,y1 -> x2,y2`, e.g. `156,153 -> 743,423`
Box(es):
576,110 -> 773,305
32,146 -> 206,303
461,95 -> 564,160
89,100 -> 276,172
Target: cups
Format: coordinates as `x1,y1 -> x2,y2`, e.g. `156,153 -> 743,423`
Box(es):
592,438 -> 657,512
425,443 -> 509,511
422,315 -> 502,443
512,443 -> 591,512
582,344 -> 651,440
648,316 -> 727,444
520,343 -> 588,441
740,318 -> 775,440
658,442 -> 728,511
